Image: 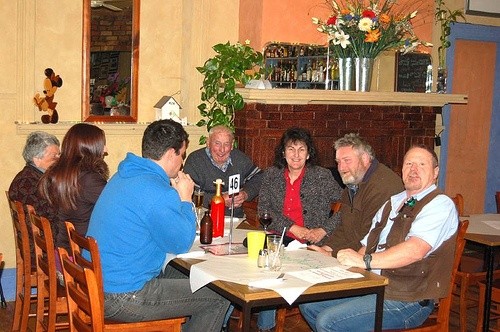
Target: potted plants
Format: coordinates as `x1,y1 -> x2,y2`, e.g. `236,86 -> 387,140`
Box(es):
195,39 -> 272,147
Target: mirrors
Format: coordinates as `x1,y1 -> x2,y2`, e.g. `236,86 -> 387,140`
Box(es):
80,0 -> 143,124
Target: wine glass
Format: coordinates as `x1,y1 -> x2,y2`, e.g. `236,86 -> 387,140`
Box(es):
257,208 -> 273,235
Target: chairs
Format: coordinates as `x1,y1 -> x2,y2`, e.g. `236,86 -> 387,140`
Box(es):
2,188 -> 191,332
393,189 -> 500,332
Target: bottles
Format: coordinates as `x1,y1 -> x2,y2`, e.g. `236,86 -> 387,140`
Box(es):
208,179 -> 226,238
200,210 -> 214,244
257,250 -> 266,268
264,41 -> 338,85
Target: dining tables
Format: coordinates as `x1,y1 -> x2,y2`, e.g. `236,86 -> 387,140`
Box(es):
167,200 -> 390,331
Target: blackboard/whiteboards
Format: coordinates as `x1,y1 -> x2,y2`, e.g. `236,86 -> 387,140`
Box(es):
395,52 -> 432,93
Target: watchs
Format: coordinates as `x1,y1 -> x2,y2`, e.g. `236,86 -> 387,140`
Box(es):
363,253 -> 372,271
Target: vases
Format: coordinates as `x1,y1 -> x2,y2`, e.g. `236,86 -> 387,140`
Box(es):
355,1 -> 422,92
306,1 -> 357,93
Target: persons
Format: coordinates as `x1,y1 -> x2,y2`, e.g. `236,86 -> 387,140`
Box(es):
304,133 -> 410,256
39,123 -> 111,285
9,130 -> 62,271
184,126 -> 265,231
81,120 -> 232,332
220,126 -> 346,332
298,143 -> 460,332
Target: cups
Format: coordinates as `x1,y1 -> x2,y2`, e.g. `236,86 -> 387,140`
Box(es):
267,235 -> 284,272
194,188 -> 206,209
247,232 -> 267,260
